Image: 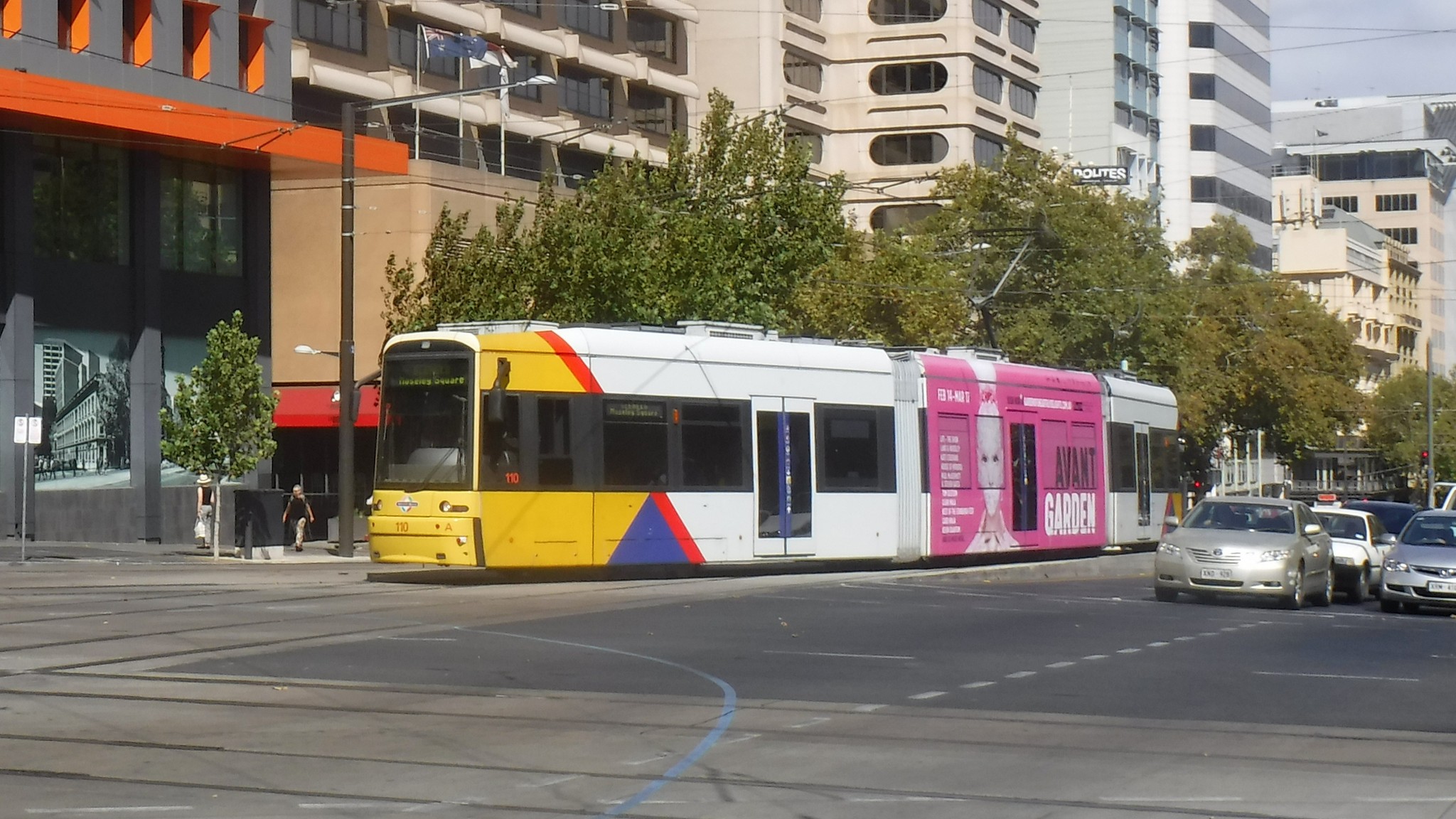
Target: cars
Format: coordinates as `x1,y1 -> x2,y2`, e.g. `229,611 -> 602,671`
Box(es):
1154,496 -> 1335,610
1304,482 -> 1456,614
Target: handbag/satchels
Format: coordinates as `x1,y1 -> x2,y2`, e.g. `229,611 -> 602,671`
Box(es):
194,516 -> 205,539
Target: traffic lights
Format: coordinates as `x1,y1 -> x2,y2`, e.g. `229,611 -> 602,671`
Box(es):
1195,481 -> 1201,488
1421,450 -> 1429,466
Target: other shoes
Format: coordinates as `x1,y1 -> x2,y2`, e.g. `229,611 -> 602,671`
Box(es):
295,545 -> 303,551
196,543 -> 210,549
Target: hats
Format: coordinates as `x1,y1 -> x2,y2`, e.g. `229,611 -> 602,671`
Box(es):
196,474 -> 211,483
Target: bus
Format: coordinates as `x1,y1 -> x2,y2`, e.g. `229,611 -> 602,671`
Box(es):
366,316 -> 1184,574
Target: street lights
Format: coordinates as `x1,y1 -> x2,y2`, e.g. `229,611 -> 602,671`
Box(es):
337,76 -> 557,558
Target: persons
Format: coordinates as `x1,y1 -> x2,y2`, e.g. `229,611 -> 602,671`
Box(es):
196,474 -> 215,549
282,485 -> 315,552
38,455 -> 56,480
72,458 -> 77,477
95,458 -> 109,476
